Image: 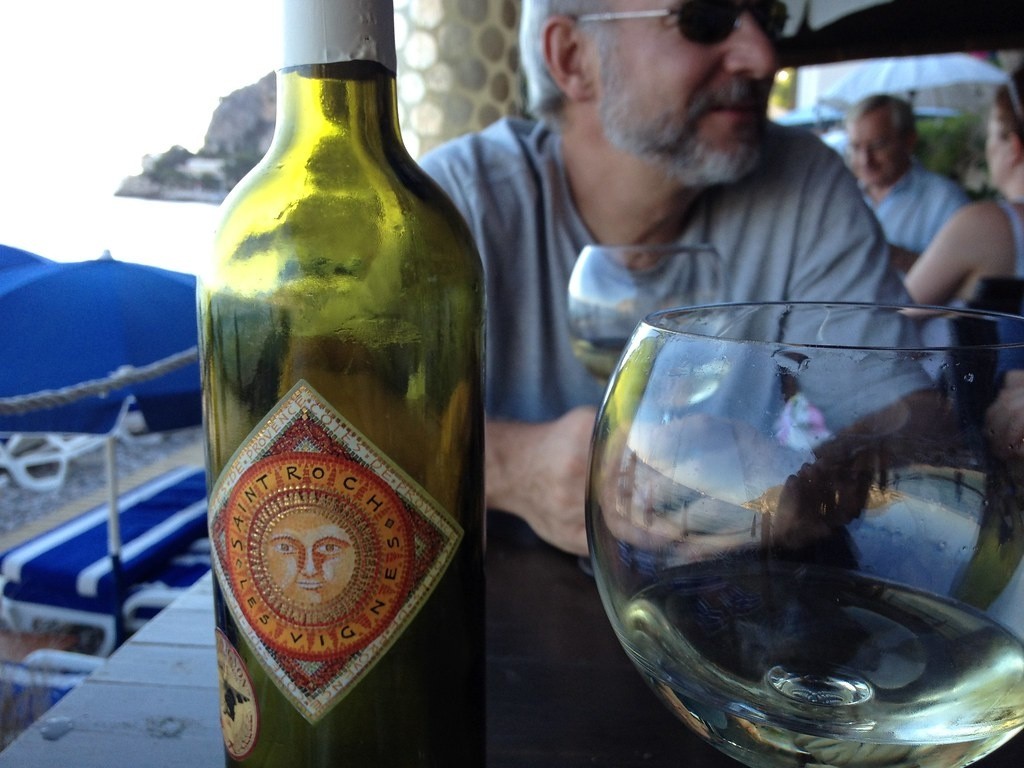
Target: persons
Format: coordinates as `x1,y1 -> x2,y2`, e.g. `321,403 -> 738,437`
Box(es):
422,1 -> 955,556
984,370 -> 1024,462
811,65 -> 1024,308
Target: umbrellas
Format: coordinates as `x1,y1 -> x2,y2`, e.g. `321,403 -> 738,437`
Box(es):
0,243 -> 289,652
778,0 -> 1024,131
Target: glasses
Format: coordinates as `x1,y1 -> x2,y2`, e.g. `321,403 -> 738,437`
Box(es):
573,0 -> 789,44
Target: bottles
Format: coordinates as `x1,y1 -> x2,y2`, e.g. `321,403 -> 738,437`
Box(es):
191,0 -> 494,768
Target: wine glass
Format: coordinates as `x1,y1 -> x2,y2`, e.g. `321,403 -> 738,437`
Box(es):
581,298 -> 1024,768
564,242 -> 733,382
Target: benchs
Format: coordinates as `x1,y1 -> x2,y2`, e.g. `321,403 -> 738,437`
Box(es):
1,465 -> 207,657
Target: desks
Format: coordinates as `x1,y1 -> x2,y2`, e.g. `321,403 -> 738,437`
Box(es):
0,509 -> 749,768
